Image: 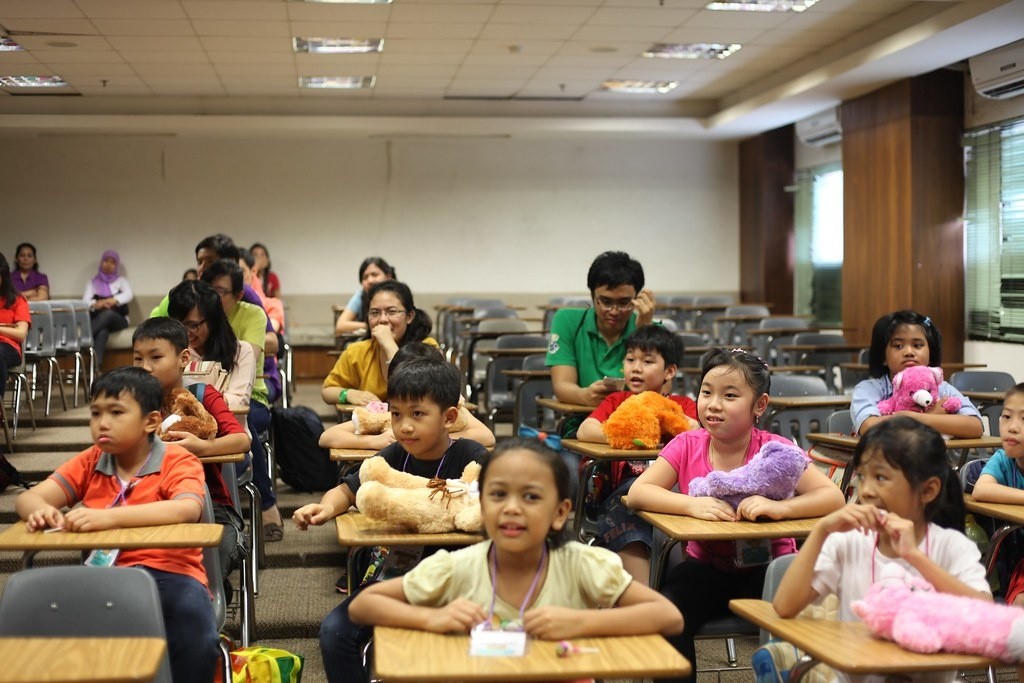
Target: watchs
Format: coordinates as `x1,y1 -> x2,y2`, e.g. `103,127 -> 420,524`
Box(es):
338,388 -> 352,405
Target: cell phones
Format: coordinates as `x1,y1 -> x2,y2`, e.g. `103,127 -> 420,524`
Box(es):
602,376 -> 626,391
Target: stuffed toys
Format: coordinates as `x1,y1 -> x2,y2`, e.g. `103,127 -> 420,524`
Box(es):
603,390 -> 699,451
687,441 -> 808,514
156,386 -> 218,442
849,578 -> 1024,663
356,455 -> 485,534
877,366 -> 961,416
353,393 -> 466,435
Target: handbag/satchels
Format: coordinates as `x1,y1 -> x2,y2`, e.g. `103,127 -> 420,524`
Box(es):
214,646 -> 305,683
89,290 -> 129,317
183,361 -> 229,396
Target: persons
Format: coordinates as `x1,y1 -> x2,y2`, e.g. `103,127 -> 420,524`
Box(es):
0,233 -> 1024,683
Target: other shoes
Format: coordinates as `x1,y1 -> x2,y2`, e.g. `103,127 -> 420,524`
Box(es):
334,568 -> 366,594
262,515 -> 284,541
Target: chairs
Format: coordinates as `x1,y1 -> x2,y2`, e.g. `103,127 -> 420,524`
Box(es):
0,300 -> 1024,683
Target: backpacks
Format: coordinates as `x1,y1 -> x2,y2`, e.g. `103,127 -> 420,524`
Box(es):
268,405 -> 340,494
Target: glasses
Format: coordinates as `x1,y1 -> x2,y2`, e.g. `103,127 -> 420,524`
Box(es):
183,318 -> 205,332
367,305 -> 408,321
594,296 -> 634,312
215,289 -> 233,297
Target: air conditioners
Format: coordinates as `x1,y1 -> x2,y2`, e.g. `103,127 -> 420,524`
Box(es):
968,37 -> 1024,102
796,107 -> 843,151
